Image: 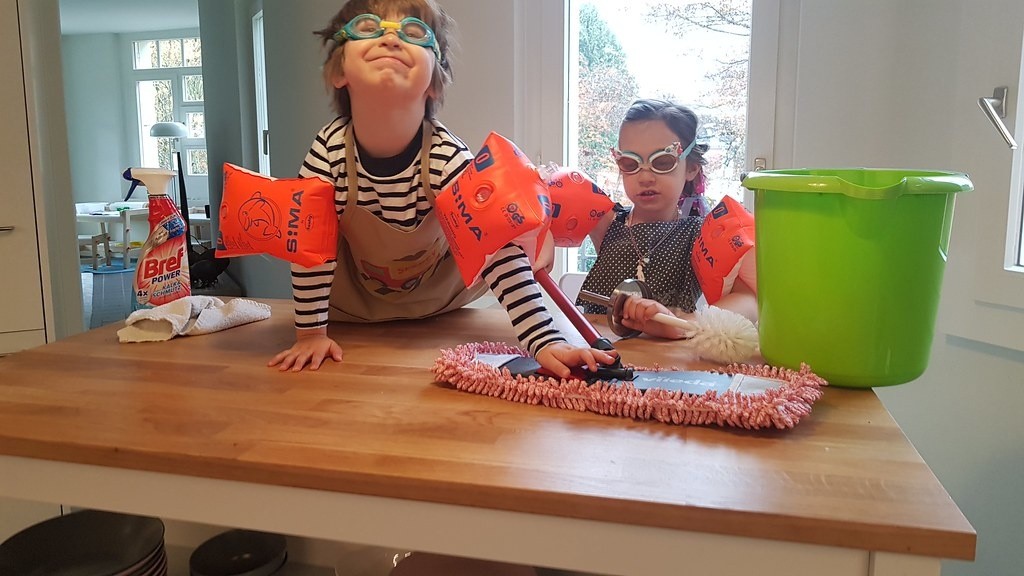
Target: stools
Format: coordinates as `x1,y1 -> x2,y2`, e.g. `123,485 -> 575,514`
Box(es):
78,232 -> 111,269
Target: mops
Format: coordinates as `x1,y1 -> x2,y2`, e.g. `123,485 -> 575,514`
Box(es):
431,268 -> 828,431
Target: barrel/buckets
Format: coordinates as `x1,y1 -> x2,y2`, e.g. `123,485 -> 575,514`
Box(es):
740,168 -> 974,387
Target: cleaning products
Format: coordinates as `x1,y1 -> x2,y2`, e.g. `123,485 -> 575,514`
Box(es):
123,166 -> 191,317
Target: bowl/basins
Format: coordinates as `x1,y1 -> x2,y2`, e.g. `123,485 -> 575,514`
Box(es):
189,529 -> 289,576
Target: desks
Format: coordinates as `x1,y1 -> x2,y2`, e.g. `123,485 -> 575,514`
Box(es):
76,208 -> 150,269
0,295 -> 978,576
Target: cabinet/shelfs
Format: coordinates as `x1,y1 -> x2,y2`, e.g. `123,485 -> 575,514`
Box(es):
0,0 -> 84,354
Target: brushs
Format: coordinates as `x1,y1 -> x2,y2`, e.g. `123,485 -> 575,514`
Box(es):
579,278 -> 760,364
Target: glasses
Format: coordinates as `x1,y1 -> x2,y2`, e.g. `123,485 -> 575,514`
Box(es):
333,13 -> 441,60
610,140 -> 696,175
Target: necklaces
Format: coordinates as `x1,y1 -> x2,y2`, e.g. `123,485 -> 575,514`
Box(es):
629,206 -> 679,282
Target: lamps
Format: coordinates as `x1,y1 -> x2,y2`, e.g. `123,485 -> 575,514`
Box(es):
150,121 -> 188,206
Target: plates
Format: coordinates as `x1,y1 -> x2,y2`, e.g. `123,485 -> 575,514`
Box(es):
0,510 -> 168,576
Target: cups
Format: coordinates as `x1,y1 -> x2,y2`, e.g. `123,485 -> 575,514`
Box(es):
205,205 -> 210,218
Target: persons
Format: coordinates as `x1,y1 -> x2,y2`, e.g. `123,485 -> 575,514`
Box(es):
268,0 -> 619,378
573,97 -> 758,339
